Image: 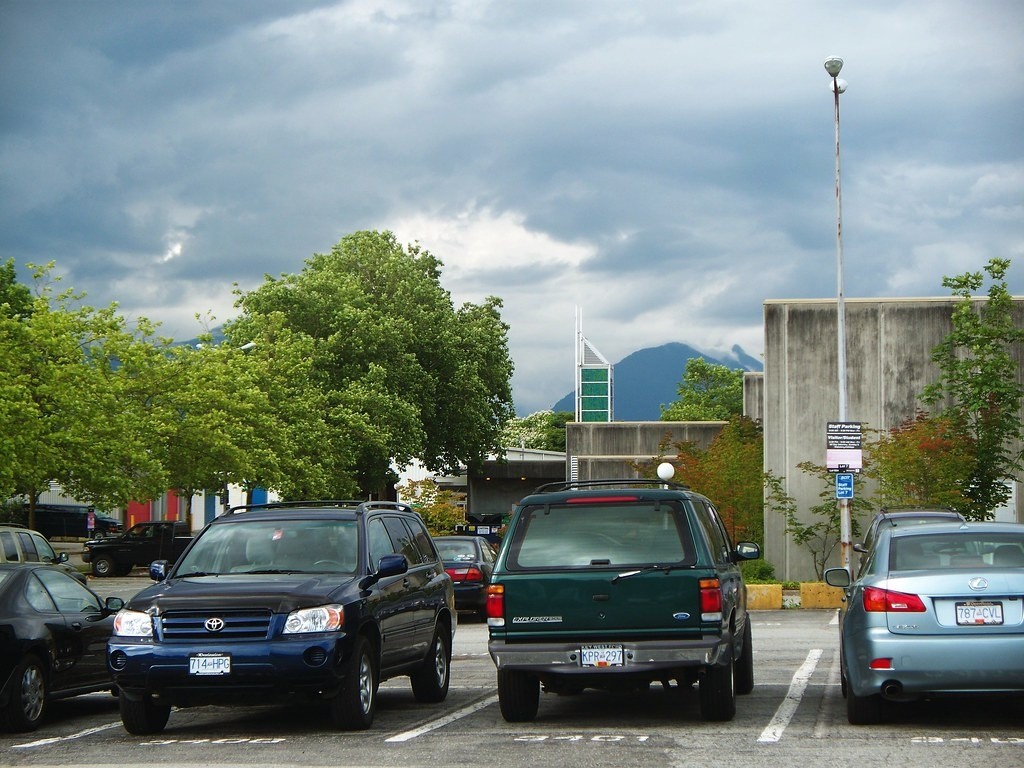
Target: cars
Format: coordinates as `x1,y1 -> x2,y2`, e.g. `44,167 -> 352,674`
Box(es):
429,535 -> 493,623
0,562 -> 124,733
823,522 -> 1024,723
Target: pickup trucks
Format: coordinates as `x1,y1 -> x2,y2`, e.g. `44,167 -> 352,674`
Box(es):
81,520 -> 223,578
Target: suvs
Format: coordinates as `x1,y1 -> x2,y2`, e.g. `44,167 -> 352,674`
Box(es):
1,521 -> 89,589
853,507 -> 996,574
110,499 -> 457,735
489,477 -> 762,726
17,502 -> 123,543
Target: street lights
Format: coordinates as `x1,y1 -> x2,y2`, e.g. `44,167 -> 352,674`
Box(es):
825,55 -> 857,586
655,460 -> 675,532
